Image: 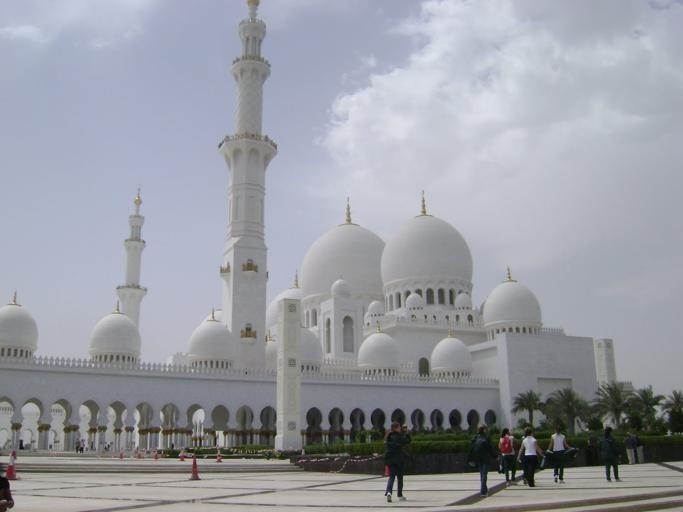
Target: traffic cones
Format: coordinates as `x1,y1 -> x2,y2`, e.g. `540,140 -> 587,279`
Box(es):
190,456 -> 201,480
115,446 -> 223,463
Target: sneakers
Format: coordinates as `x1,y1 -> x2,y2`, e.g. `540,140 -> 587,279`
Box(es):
387,493 -> 391,502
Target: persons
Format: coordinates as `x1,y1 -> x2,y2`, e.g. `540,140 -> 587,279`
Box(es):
468,425 -> 499,497
384,421 -> 411,502
516,426 -> 644,487
75,439 -> 89,453
0,475 -> 14,512
498,427 -> 518,487
